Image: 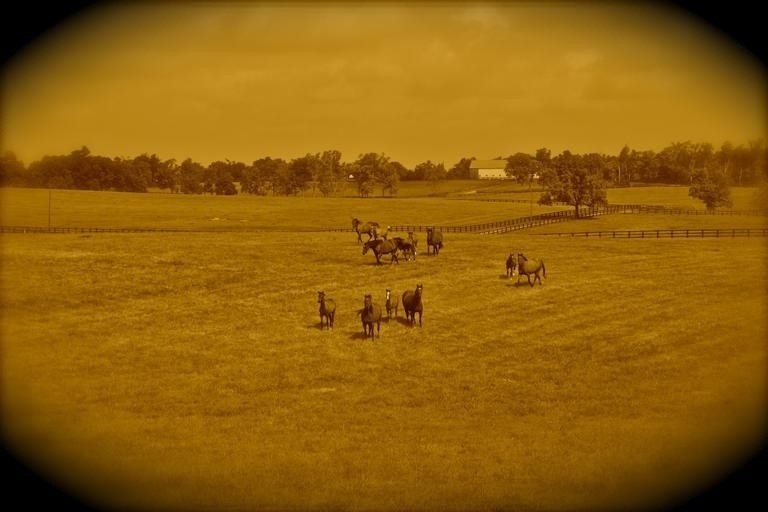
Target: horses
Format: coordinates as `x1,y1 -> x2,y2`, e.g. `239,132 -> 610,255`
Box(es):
354,295 -> 382,342
386,289 -> 399,328
352,218 -> 419,265
518,253 -> 546,287
402,284 -> 423,327
426,228 -> 443,256
317,291 -> 336,330
506,252 -> 516,278
